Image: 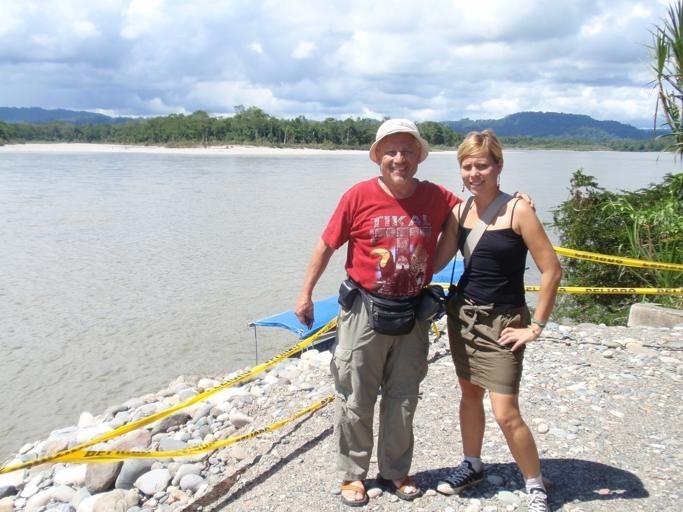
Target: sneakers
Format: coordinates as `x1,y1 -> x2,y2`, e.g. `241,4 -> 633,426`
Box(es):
528,488 -> 549,512
437,461 -> 484,494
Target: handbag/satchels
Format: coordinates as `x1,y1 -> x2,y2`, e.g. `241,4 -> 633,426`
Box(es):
418,285 -> 446,322
361,291 -> 415,335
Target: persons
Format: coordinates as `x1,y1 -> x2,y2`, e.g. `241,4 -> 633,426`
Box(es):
433,128 -> 563,511
294,117 -> 537,508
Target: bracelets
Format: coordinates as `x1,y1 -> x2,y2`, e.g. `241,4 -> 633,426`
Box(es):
527,325 -> 538,336
530,319 -> 545,328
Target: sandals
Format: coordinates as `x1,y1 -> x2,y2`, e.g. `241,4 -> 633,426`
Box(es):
377,472 -> 420,502
341,478 -> 369,505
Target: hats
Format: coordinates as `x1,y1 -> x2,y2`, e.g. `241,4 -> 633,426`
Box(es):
369,120 -> 429,163
